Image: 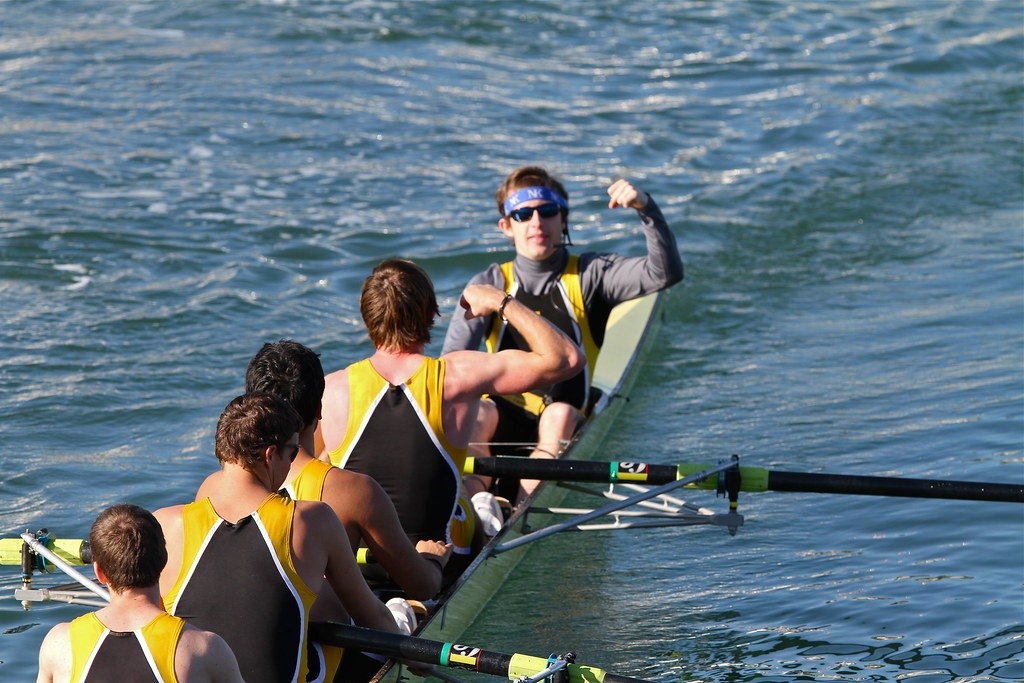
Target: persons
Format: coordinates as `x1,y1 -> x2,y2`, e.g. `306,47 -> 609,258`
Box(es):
152,390 -> 420,683
35,503 -> 247,683
194,339 -> 454,628
318,258 -> 587,584
439,165 -> 685,515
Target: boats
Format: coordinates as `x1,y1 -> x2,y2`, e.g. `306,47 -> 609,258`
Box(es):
372,286 -> 664,683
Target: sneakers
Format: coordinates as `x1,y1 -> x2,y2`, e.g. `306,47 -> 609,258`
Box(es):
384,598 -> 417,636
470,492 -> 503,538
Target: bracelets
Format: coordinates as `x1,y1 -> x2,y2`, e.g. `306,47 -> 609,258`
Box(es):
424,557 -> 443,574
499,294 -> 512,322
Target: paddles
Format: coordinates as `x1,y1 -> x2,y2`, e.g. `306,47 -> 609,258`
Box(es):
303,616 -> 664,683
0,534 -> 96,568
458,447 -> 1024,504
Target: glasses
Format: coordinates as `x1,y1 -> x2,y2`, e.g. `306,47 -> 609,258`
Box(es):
508,202 -> 562,223
282,444 -> 300,462
